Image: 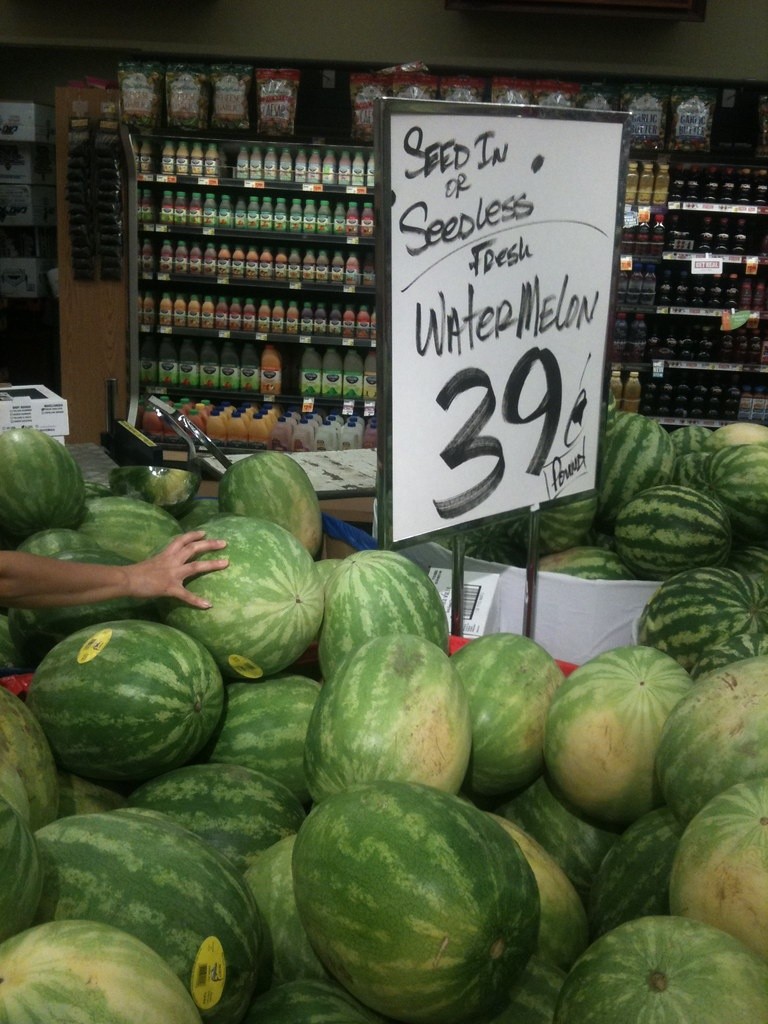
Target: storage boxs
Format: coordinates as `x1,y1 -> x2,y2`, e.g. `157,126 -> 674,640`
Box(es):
1,385 -> 71,447
0,103 -> 58,302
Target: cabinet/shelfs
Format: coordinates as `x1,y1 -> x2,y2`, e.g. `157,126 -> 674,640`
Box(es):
120,121 -> 768,463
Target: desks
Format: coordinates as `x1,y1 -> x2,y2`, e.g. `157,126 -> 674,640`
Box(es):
157,448 -> 378,523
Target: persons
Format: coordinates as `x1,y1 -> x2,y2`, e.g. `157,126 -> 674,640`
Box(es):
0,532 -> 229,608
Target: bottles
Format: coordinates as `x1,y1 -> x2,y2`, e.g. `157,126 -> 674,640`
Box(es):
136,141 -> 766,449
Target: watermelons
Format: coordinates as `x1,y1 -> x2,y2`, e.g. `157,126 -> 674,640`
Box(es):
0,407 -> 767,1024
